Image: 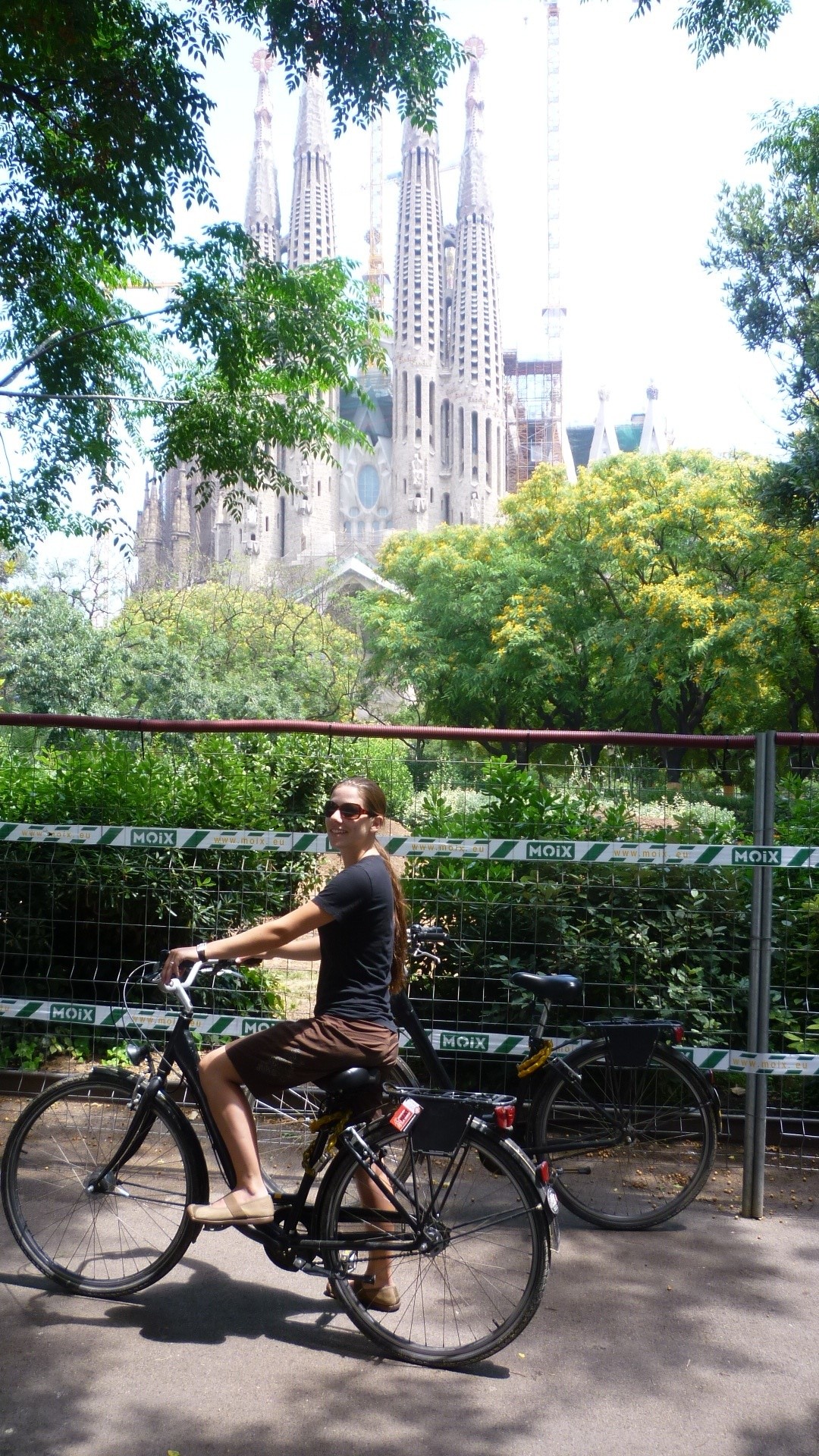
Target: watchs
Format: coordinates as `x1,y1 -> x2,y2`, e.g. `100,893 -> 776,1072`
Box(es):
197,942 -> 209,963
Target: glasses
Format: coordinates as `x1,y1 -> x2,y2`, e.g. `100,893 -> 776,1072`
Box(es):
324,801 -> 376,820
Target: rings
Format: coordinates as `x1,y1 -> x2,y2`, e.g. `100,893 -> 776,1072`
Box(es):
169,962 -> 174,965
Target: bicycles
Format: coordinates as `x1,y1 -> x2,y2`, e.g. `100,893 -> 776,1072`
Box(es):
2,958 -> 561,1372
393,922 -> 721,1231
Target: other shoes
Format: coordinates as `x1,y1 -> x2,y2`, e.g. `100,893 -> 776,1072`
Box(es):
188,1192 -> 274,1223
326,1281 -> 400,1310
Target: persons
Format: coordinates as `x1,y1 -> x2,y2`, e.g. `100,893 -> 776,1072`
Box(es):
161,777 -> 406,1311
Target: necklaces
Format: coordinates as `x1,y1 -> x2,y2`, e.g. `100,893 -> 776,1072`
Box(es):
357,841 -> 372,863
